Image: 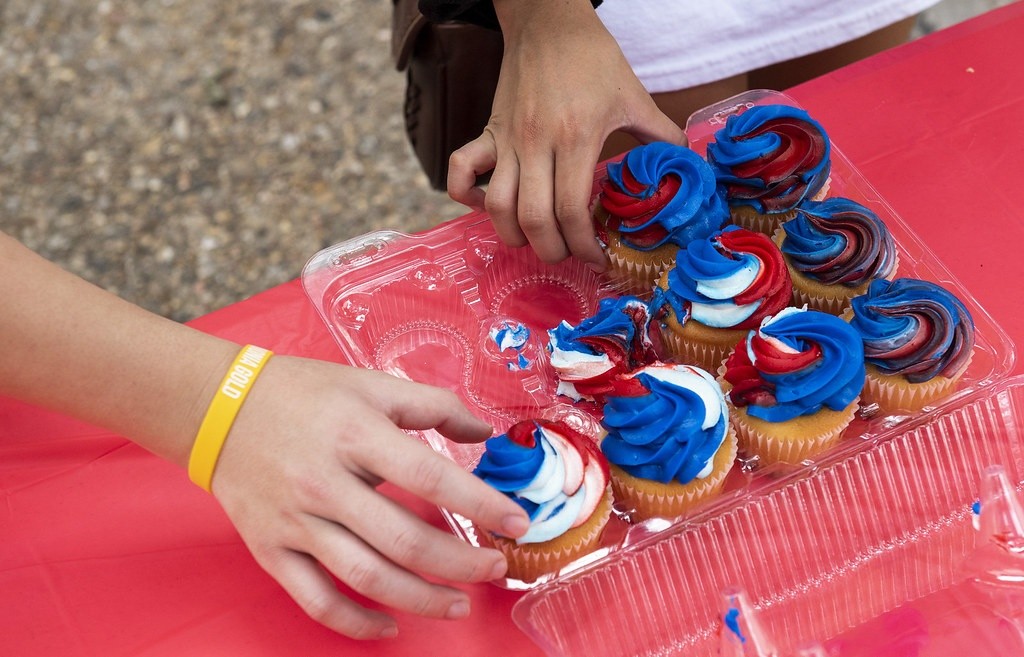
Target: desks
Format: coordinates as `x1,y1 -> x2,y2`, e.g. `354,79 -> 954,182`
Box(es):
0,0 -> 1024,657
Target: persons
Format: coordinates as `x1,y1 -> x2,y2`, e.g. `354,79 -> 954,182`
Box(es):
447,0 -> 948,269
1,232 -> 531,644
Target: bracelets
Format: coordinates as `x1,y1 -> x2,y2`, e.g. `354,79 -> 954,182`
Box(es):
187,342 -> 273,494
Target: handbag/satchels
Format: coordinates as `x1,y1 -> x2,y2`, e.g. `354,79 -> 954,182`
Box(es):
392,0 -> 505,191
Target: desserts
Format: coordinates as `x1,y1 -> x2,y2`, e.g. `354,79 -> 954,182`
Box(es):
466,104 -> 975,582
